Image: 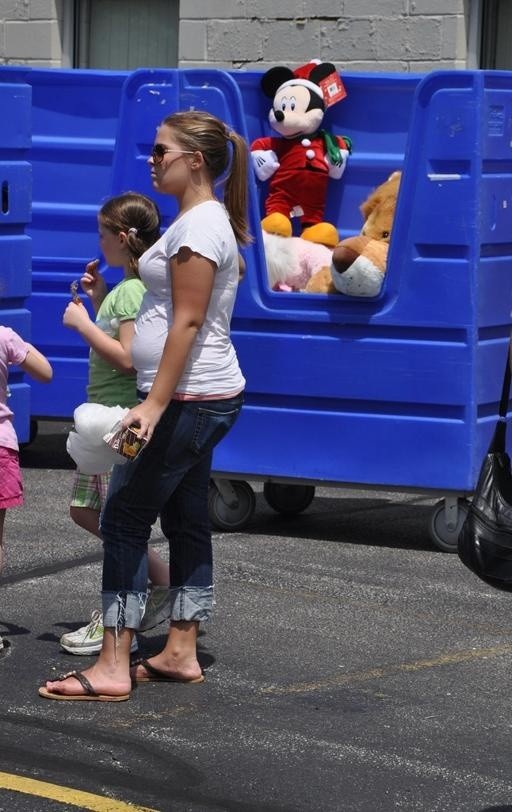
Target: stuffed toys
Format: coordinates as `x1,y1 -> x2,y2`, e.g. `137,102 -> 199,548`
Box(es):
259,228 -> 336,296
248,57 -> 353,254
331,171 -> 404,299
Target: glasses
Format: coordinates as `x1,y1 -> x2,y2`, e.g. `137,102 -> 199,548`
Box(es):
153,145 -> 195,165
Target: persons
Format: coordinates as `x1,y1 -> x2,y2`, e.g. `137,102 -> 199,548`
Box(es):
37,107 -> 255,704
0,325 -> 56,549
58,191 -> 178,656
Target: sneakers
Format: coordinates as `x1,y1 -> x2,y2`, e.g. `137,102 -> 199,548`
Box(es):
137,585 -> 172,632
61,616 -> 139,655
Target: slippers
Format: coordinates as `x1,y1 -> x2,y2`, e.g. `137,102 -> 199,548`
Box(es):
38,669 -> 131,701
130,658 -> 204,683
